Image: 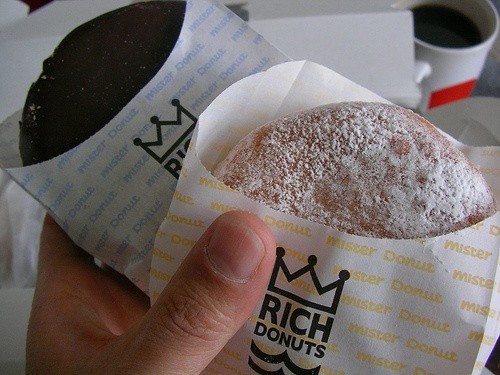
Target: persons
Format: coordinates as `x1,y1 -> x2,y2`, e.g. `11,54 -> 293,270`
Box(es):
27,208 -> 277,373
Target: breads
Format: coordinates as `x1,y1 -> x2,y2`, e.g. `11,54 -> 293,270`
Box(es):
209,104 -> 499,239
20,0 -> 190,166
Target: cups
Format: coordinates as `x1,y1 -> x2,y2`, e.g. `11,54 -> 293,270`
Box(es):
390,1 -> 500,114
